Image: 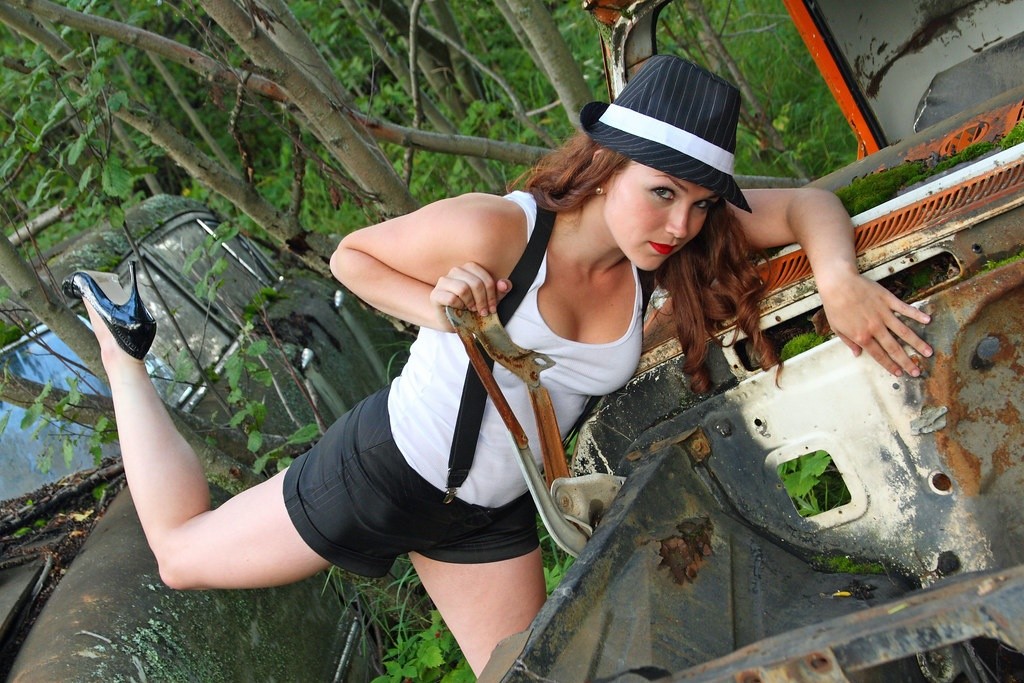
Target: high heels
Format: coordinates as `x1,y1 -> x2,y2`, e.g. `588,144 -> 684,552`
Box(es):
61,261 -> 156,361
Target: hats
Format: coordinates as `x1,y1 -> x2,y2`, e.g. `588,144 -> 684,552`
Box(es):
579,56 -> 752,215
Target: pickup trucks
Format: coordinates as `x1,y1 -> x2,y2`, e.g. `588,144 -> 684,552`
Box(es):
479,0 -> 1024,683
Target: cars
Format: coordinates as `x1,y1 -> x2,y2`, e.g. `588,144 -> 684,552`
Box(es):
0,194 -> 418,683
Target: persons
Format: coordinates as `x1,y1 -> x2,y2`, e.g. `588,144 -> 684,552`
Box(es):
62,54 -> 932,679
